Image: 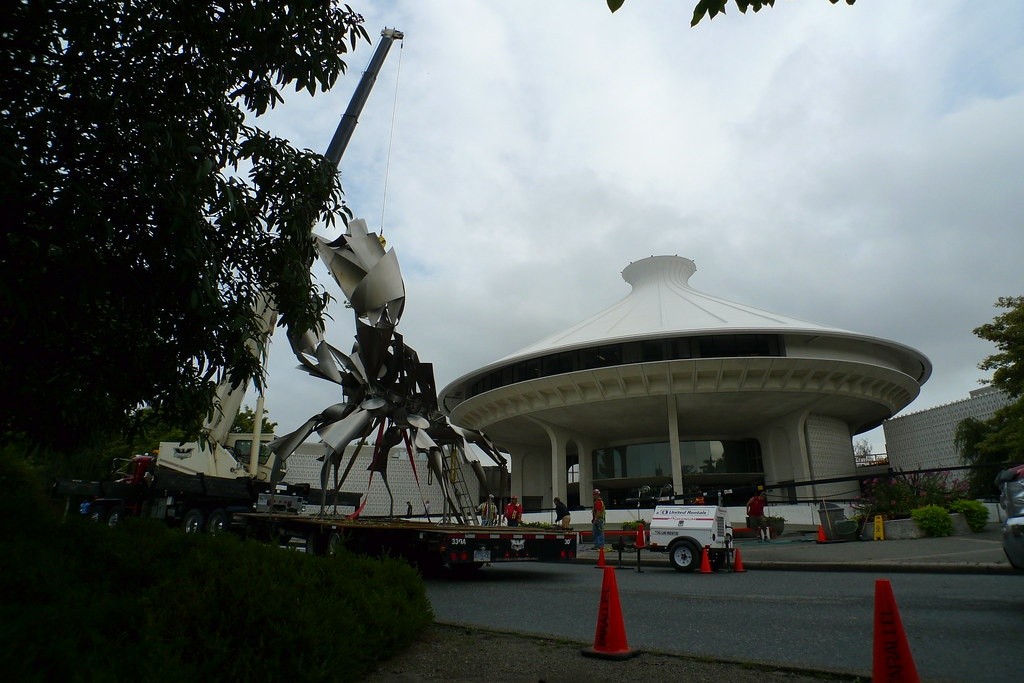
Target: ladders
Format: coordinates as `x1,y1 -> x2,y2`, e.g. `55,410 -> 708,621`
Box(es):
441,443 -> 480,526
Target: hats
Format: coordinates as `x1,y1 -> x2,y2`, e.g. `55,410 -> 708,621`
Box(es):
552,498 -> 559,501
511,495 -> 517,499
592,489 -> 600,496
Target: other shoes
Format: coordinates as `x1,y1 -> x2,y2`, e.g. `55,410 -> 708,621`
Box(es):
764,538 -> 770,542
590,547 -> 597,550
758,539 -> 762,542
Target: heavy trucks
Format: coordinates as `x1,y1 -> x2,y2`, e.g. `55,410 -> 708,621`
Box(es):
86,454 -> 579,580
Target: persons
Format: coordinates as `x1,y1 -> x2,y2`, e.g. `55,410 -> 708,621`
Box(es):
425,499 -> 431,513
693,491 -> 704,504
552,497 -> 571,528
474,494 -> 498,528
745,492 -> 771,543
406,501 -> 412,515
501,496 -> 522,527
591,488 -> 606,550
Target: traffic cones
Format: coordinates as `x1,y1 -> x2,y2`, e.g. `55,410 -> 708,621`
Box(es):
816,524 -> 827,543
594,548 -> 607,569
580,565 -> 641,662
870,578 -> 924,683
633,524 -> 647,548
733,547 -> 748,573
698,548 -> 714,574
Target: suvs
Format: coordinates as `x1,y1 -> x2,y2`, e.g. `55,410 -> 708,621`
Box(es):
995,464 -> 1024,572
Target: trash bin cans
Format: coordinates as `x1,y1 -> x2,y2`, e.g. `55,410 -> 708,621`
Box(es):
818,502 -> 845,539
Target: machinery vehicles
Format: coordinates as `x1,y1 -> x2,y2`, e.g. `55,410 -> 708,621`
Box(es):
141,28 -> 406,520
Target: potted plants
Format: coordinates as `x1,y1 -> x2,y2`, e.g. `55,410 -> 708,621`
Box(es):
763,514 -> 790,536
620,519 -> 650,540
